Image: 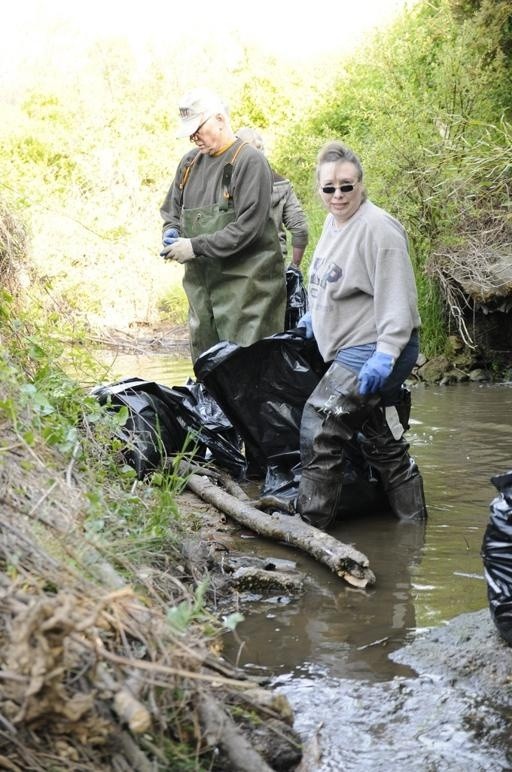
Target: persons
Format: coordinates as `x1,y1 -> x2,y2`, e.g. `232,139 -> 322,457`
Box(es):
159,100 -> 290,365
234,125 -> 309,269
297,140 -> 431,529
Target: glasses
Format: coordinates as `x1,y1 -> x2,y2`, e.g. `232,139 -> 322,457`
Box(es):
318,178 -> 358,192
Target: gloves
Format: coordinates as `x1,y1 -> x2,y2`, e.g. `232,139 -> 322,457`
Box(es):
358,352 -> 395,396
297,310 -> 313,339
161,229 -> 196,264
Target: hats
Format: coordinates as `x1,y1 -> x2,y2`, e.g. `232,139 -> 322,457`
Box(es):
175,90 -> 224,139
